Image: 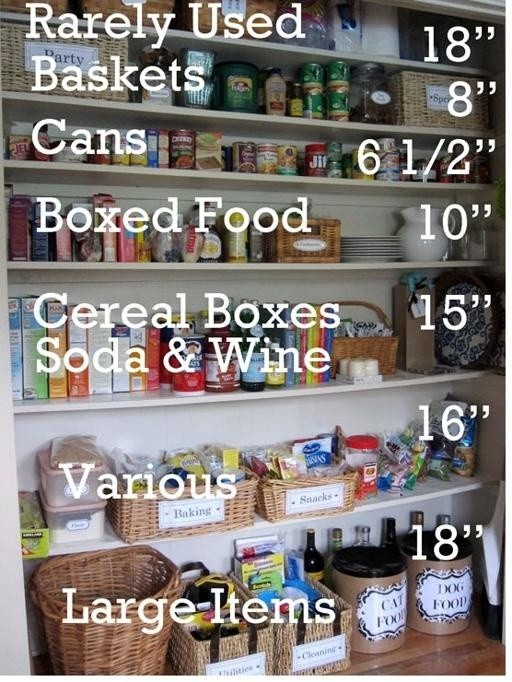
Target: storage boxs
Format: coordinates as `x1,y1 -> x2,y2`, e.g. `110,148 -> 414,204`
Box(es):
0,10 -> 501,559
38,442 -> 110,545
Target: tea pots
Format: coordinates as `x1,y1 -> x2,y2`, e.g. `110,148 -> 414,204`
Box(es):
396,205 -> 455,262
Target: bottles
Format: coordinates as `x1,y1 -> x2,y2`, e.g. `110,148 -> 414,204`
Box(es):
153,202 -> 266,263
297,510 -> 453,591
345,435 -> 379,501
162,297 -> 286,396
349,64 -> 391,124
454,192 -> 503,261
265,68 -> 286,115
33,121 -> 51,162
289,81 -> 304,117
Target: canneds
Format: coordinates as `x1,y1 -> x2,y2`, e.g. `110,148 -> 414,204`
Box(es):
203,335 -> 241,392
171,334 -> 205,396
88,60 -> 489,183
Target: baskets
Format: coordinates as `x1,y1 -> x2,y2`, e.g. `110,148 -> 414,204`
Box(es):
30,544 -> 183,675
102,461 -> 261,544
253,454 -> 359,523
0,0 -> 276,40
327,300 -> 399,376
1,22 -> 130,104
261,212 -> 342,264
385,71 -> 490,130
168,569 -> 352,675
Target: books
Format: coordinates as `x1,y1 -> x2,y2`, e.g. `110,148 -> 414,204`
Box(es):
284,304 -> 334,387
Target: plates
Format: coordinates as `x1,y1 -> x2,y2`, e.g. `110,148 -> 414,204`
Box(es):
339,235 -> 403,262
